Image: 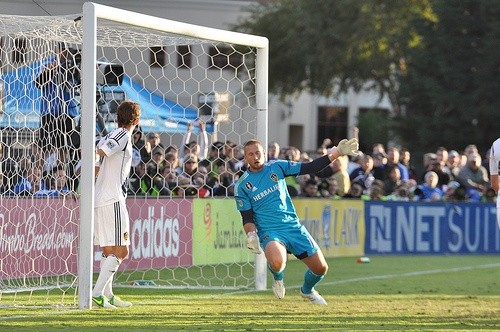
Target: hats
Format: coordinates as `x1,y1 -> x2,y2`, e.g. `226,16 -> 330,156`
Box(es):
184,153 -> 197,163
152,148 -> 163,155
468,154 -> 481,161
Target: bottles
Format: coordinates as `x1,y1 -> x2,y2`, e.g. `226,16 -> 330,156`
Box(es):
357,256 -> 369,263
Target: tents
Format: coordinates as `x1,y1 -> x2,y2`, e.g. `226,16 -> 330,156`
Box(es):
0,55 -> 218,142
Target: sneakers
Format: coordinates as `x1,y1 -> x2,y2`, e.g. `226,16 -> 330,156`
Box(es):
272,278 -> 286,299
300,287 -> 327,305
108,296 -> 132,307
92,296 -> 117,309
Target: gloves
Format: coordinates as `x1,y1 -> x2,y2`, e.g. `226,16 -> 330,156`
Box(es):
336,138 -> 358,157
246,231 -> 262,254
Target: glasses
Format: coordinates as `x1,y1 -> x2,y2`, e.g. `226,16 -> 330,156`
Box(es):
372,154 -> 385,160
150,137 -> 159,140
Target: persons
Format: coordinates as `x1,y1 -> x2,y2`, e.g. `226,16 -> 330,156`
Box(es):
35,48 -> 82,191
234,138 -> 359,306
490,138 -> 500,230
0,120 -> 497,203
91,101 -> 140,307
